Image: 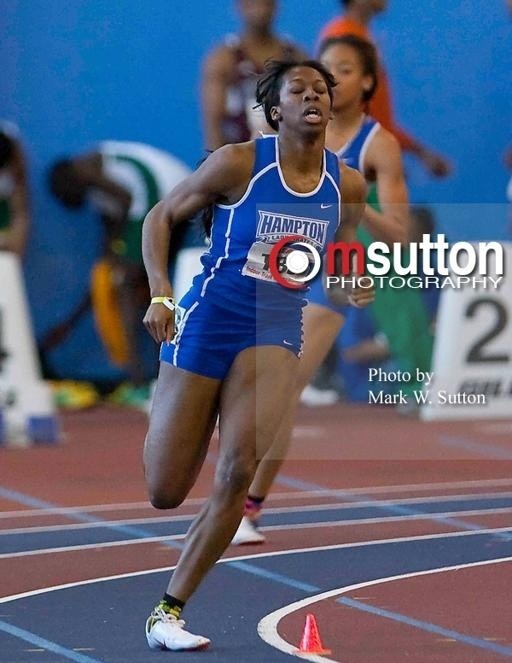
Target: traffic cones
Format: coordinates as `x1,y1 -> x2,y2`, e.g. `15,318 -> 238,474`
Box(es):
290,612 -> 332,656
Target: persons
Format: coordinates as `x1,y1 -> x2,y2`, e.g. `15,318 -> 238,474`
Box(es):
301,205 -> 450,410
141,55 -> 379,651
318,1 -> 453,179
201,0 -> 308,152
231,32 -> 412,547
48,138 -> 195,405
1,121 -> 32,257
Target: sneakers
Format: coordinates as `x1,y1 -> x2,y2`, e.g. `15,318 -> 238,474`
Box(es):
144,614 -> 211,651
342,337 -> 393,363
24,413 -> 62,445
231,514 -> 268,547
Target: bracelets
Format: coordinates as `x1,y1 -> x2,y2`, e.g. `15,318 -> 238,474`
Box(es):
151,295 -> 177,308
